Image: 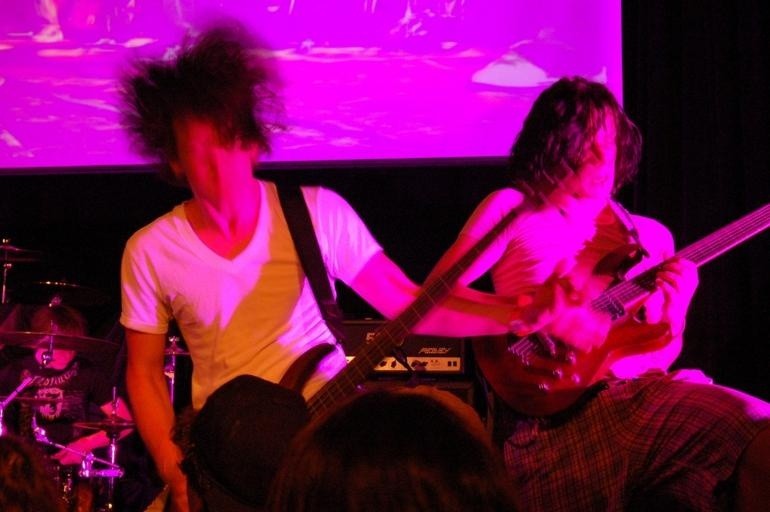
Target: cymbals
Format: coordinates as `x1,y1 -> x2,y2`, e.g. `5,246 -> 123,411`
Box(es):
2,329 -> 109,350
0,243 -> 36,262
72,418 -> 138,431
160,345 -> 191,357
28,277 -> 89,290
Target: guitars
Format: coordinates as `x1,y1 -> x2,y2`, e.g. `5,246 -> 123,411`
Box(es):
470,202 -> 770,417
114,143 -> 603,512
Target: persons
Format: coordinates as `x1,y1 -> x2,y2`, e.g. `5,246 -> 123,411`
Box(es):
167,368 -> 310,512
116,21 -> 568,512
1,302 -> 136,512
262,379 -> 527,512
418,70 -> 770,511
